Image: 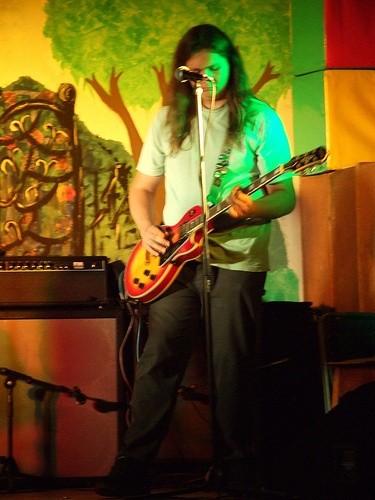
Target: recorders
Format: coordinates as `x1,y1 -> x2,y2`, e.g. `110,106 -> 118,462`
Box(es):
0,252 -> 111,307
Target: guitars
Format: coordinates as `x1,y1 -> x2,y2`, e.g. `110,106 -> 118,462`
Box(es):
124,144 -> 332,304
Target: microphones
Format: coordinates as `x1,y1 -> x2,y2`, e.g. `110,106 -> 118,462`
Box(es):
58,83 -> 73,102
175,65 -> 214,83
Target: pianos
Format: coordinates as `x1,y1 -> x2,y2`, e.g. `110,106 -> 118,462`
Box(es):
0,252 -> 111,308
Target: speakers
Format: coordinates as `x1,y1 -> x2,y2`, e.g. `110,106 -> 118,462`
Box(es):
1,309 -> 125,485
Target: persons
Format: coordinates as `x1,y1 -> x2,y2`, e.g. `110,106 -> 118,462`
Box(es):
91,22 -> 297,500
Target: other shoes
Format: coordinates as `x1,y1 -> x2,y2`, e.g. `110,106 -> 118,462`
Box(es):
95,456 -> 152,497
205,452 -> 253,485
